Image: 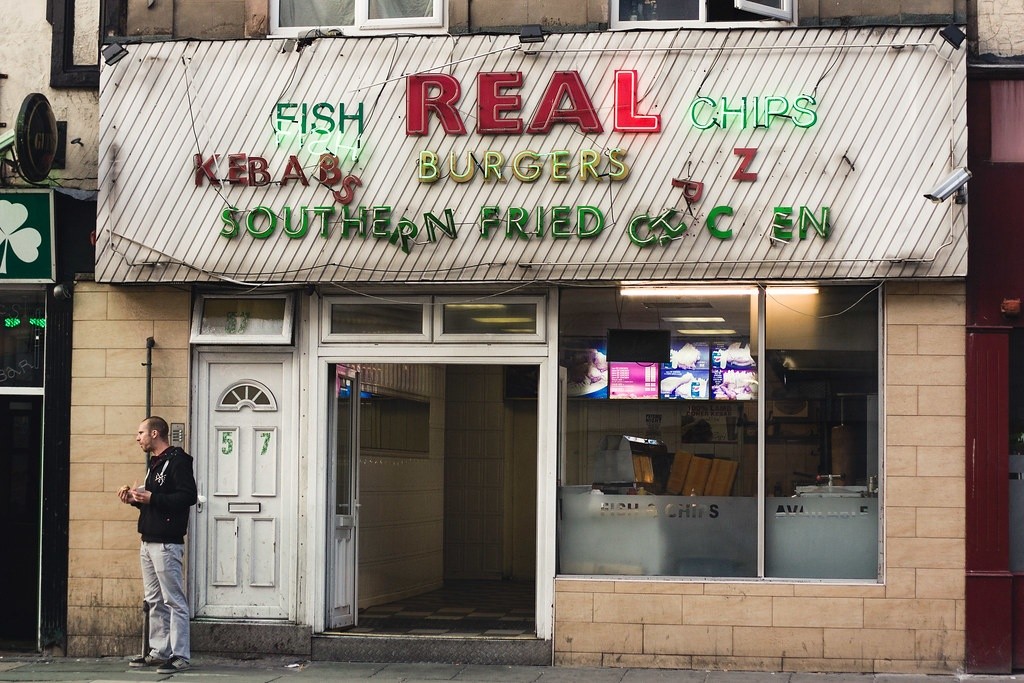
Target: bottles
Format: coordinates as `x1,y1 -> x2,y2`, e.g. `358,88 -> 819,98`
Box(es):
690,488 -> 696,507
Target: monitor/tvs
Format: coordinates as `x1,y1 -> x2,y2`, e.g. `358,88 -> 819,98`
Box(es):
605,329 -> 673,363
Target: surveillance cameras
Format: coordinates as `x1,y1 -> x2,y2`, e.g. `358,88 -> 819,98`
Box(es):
922,167 -> 972,203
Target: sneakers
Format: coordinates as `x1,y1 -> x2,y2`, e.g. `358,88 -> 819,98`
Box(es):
157,656 -> 191,672
129,653 -> 167,666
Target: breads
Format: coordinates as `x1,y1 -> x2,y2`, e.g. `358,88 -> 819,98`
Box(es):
632,455 -> 653,482
666,450 -> 737,496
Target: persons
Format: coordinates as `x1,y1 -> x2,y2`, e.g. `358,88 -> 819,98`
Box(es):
117,416 -> 198,673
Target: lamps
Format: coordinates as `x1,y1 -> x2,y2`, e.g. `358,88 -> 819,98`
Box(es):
519,24 -> 551,45
938,25 -> 980,51
99,38 -> 131,69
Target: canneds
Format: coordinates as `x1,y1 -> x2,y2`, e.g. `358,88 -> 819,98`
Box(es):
712,352 -> 721,368
691,381 -> 700,397
